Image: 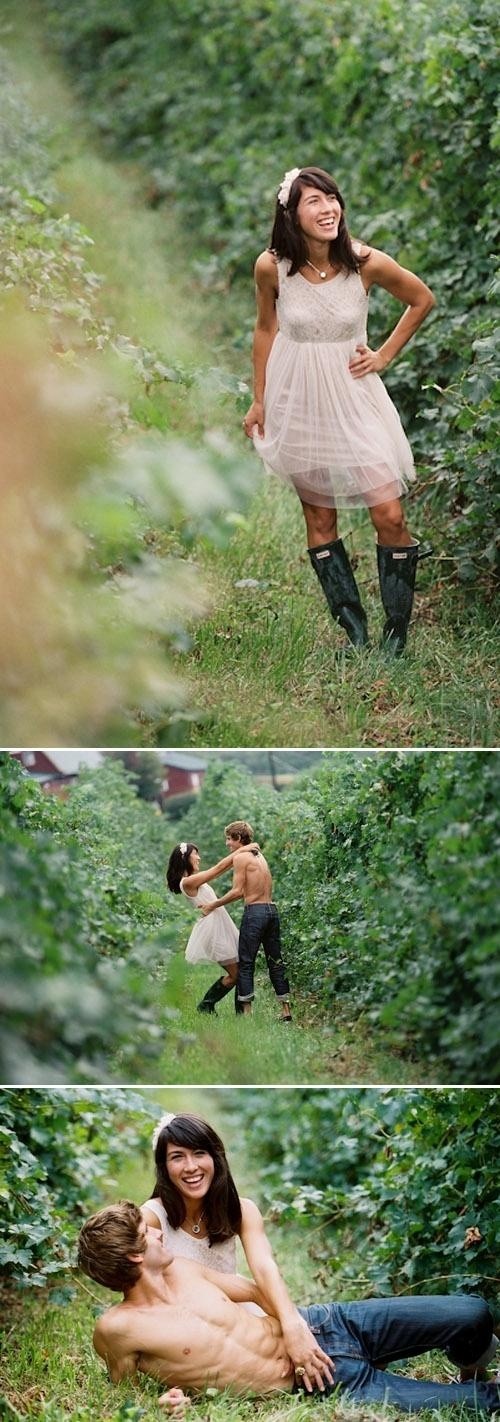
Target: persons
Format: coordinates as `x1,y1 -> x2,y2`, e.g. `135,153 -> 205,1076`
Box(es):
137,1113 -> 337,1393
196,821 -> 294,1022
77,1197 -> 500,1421
166,839 -> 261,1016
239,167 -> 436,672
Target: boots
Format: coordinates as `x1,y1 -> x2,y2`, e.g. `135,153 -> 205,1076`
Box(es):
197,976 -> 230,1017
234,985 -> 243,1014
375,538 -> 420,651
307,538 -> 372,659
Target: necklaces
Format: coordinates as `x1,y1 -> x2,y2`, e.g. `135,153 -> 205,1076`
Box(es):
306,259 -> 331,279
184,1210 -> 204,1234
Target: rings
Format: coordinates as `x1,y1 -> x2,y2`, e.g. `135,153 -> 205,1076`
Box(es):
240,420 -> 246,429
295,1366 -> 305,1376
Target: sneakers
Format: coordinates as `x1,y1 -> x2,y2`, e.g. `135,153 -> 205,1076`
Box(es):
280,1015 -> 292,1021
